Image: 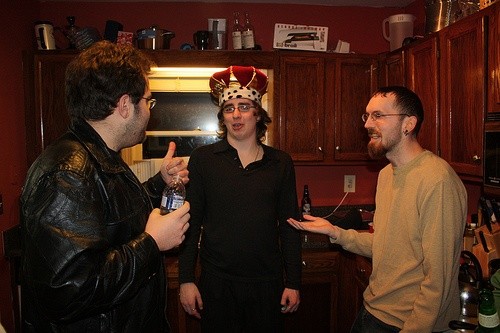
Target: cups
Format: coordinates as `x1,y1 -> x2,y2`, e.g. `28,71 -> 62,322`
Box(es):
208,18 -> 226,49
193,30 -> 210,50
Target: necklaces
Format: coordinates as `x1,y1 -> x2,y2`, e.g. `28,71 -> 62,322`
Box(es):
254,145 -> 260,161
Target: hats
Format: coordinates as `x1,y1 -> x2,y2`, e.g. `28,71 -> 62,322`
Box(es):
209,66 -> 269,107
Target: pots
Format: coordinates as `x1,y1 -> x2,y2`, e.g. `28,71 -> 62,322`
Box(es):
136,25 -> 176,50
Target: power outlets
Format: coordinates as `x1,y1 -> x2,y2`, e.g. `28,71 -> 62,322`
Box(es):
344,175 -> 356,193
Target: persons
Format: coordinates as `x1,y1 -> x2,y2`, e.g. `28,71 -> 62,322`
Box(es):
287,85 -> 467,333
180,66 -> 301,333
21,40 -> 191,333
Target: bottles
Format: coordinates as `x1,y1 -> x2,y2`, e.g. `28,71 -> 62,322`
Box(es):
477,292 -> 500,333
160,172 -> 186,215
302,185 -> 312,216
64,16 -> 81,50
233,16 -> 243,51
242,13 -> 255,51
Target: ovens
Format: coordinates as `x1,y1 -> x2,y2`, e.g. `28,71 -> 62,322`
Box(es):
130,66 -> 270,161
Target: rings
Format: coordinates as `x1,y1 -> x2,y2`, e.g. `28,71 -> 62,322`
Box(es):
191,308 -> 196,312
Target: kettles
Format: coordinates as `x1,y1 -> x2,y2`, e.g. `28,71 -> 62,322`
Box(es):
34,20 -> 56,53
458,251 -> 484,317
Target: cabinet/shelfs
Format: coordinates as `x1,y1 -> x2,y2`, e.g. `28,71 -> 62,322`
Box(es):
168,249 -> 373,333
277,0 -> 500,183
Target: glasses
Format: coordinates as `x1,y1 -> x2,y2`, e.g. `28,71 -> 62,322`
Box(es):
222,105 -> 258,114
361,112 -> 410,122
115,95 -> 156,109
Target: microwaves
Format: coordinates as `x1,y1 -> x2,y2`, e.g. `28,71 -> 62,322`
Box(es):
483,121 -> 500,189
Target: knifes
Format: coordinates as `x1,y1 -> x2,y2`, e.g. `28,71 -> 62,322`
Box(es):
479,196 -> 500,253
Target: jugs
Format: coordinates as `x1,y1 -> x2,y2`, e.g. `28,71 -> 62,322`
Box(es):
382,14 -> 416,52
